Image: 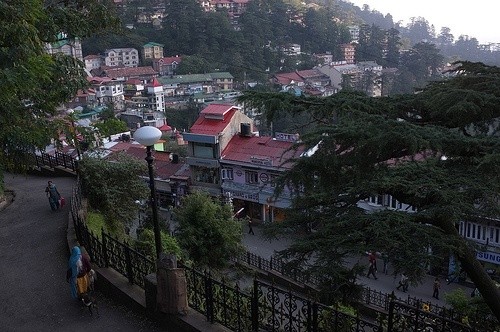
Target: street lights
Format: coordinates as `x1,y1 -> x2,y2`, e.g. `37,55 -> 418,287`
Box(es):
133,125 -> 163,278
67,109 -> 82,161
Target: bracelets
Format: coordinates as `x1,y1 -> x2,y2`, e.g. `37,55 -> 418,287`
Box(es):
477,293 -> 479,294
475,291 -> 476,292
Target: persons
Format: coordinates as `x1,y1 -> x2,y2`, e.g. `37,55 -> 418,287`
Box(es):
68,246 -> 92,297
367,255 -> 388,280
247,215 -> 255,237
45,179 -> 62,213
470,287 -> 479,298
397,272 -> 409,293
432,278 -> 440,300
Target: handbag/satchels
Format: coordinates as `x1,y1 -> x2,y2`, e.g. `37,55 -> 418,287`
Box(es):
67,268 -> 73,283
58,196 -> 65,208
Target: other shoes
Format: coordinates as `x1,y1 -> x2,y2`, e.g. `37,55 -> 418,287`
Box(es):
367,275 -> 370,278
375,278 -> 378,280
403,290 -> 406,293
432,295 -> 436,297
397,287 -> 399,290
406,289 -> 408,291
436,296 -> 438,299
